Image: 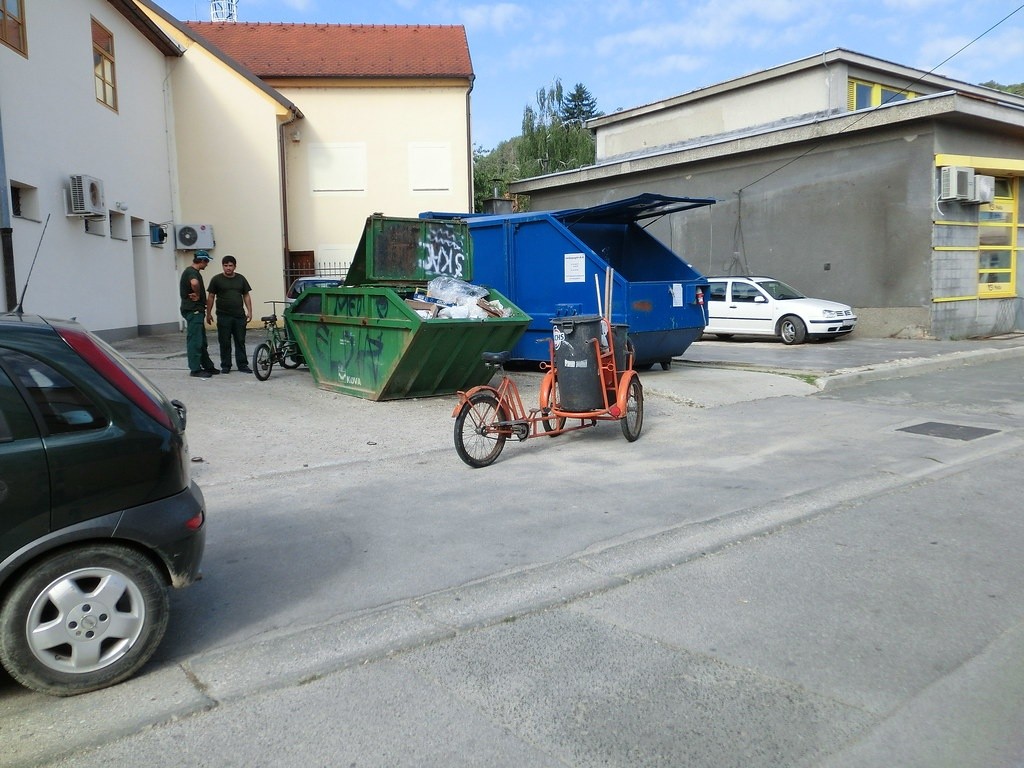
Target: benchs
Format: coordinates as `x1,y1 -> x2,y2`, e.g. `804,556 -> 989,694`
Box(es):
709,288 -> 726,301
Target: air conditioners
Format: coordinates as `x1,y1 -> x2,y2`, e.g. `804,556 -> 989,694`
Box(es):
175,224 -> 215,249
69,175 -> 106,217
941,167 -> 975,202
961,175 -> 996,205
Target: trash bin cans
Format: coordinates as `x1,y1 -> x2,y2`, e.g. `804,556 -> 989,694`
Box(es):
550,314 -> 603,412
283,213 -> 534,402
418,192 -> 716,371
607,324 -> 631,408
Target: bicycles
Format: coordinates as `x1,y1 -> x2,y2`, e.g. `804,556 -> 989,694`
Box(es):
252,300 -> 303,381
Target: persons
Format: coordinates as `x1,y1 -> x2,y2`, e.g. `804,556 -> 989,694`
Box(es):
180,250 -> 220,377
207,256 -> 253,374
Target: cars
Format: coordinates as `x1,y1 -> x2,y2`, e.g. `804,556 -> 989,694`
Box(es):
692,275 -> 857,345
285,276 -> 354,310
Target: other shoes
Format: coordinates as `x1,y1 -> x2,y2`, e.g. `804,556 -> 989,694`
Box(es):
205,367 -> 220,374
221,368 -> 229,373
190,370 -> 212,377
238,366 -> 252,374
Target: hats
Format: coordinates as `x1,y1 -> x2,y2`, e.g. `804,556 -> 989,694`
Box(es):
194,250 -> 213,261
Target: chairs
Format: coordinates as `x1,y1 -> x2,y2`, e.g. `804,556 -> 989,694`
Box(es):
733,290 -> 743,302
746,289 -> 757,303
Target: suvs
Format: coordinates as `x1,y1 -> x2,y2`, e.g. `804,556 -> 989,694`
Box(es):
0,311 -> 208,695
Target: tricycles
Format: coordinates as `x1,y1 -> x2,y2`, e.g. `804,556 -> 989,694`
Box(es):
452,316 -> 645,469
